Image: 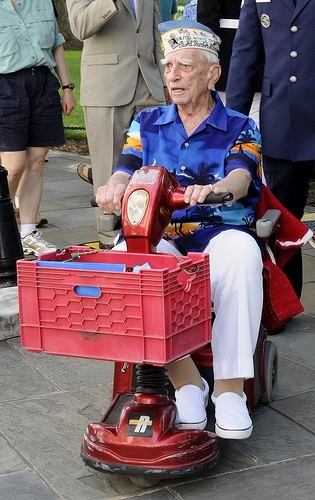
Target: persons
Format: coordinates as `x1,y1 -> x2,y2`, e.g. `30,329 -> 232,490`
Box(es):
95,19 -> 264,439
65,1 -> 168,250
223,0 -> 315,333
159,0 -> 262,95
0,0 -> 94,255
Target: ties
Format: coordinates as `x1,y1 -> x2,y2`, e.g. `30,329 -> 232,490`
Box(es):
128,0 -> 138,21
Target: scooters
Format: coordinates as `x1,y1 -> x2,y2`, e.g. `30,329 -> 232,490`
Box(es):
16,166 -> 282,488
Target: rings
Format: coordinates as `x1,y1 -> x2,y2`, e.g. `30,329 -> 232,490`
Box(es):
208,184 -> 213,191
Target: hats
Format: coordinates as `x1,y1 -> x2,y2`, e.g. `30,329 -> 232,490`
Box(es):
157,20 -> 222,57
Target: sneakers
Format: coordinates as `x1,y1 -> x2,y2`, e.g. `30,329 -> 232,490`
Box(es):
19,230 -> 60,257
172,377 -> 254,440
16,205 -> 48,227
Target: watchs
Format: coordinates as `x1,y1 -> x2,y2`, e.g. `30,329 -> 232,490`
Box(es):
62,83 -> 74,90
